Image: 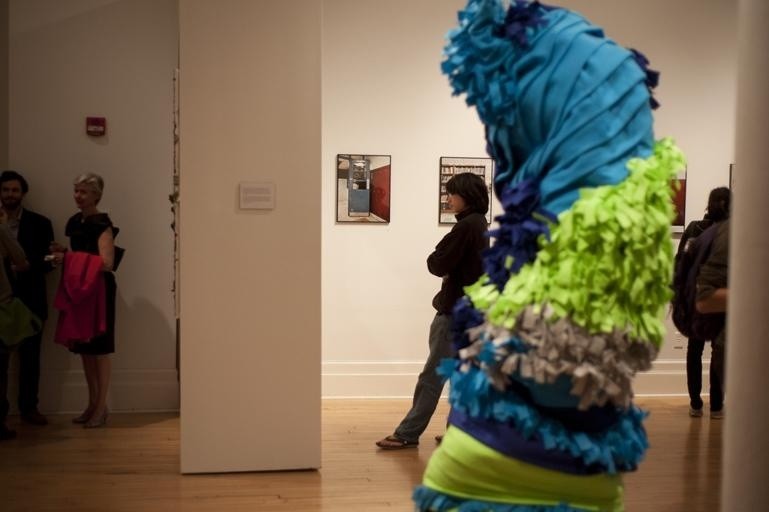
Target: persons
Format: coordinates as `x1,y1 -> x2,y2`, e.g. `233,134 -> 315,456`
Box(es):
45,171 -> 123,428
693,211 -> 728,345
681,187 -> 735,418
0,169 -> 57,425
376,173 -> 497,452
1,211 -> 42,439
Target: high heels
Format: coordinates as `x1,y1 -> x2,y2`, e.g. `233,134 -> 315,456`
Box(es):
80,406 -> 110,428
72,407 -> 93,424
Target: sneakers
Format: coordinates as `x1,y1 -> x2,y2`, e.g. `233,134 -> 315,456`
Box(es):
688,405 -> 704,419
0,423 -> 18,441
17,406 -> 49,427
710,408 -> 724,420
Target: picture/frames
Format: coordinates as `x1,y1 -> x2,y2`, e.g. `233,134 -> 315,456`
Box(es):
729,163 -> 735,190
435,155 -> 495,227
334,151 -> 394,227
665,161 -> 689,237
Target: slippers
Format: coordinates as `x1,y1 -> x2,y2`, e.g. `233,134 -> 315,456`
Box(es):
374,434 -> 420,451
